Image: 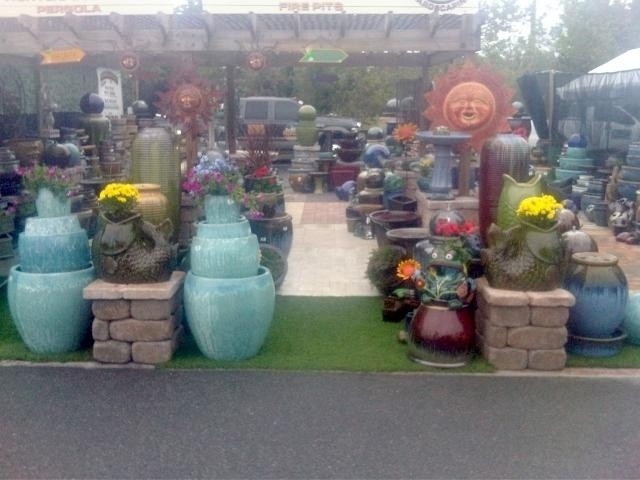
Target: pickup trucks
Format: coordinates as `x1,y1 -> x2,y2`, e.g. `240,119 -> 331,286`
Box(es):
199,97 -> 367,162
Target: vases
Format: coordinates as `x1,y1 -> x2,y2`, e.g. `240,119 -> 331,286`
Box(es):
1,93 -> 640,367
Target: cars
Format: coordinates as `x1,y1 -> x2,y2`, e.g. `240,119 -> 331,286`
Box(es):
529,103 -> 640,149
